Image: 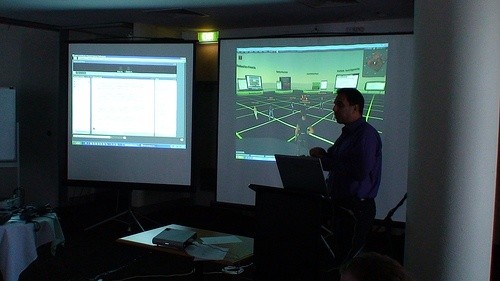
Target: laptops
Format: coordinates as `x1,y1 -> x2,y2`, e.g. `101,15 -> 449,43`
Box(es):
274,154 -> 337,199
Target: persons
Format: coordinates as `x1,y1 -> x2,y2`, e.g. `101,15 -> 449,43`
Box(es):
339,252 -> 416,281
310,88 -> 382,245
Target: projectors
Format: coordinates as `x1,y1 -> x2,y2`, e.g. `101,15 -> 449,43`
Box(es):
152,227 -> 198,249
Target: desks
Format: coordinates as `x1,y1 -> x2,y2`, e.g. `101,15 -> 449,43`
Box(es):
118,223 -> 255,281
0,212 -> 67,281
